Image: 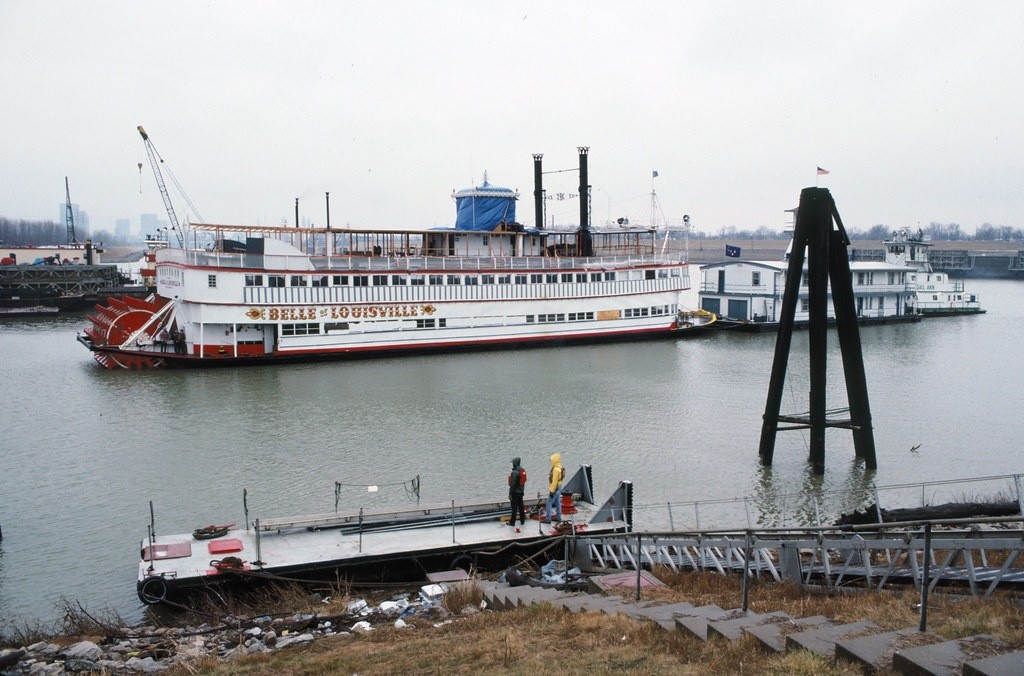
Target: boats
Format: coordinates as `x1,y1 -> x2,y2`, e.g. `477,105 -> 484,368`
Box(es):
696,257 -> 925,326
133,466 -> 636,605
879,226 -> 986,314
76,123 -> 719,374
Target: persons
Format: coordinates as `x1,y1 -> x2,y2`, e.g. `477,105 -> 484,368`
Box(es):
539,453 -> 563,524
159,327 -> 169,352
506,457 -> 527,526
172,329 -> 186,355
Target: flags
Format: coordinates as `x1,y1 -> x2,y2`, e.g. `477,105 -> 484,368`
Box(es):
726,244 -> 741,257
652,171 -> 658,177
816,166 -> 830,175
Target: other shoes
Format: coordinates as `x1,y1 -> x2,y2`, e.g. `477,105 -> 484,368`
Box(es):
540,520 -> 551,524
506,523 -> 515,526
521,521 -> 524,524
551,518 -> 561,522
